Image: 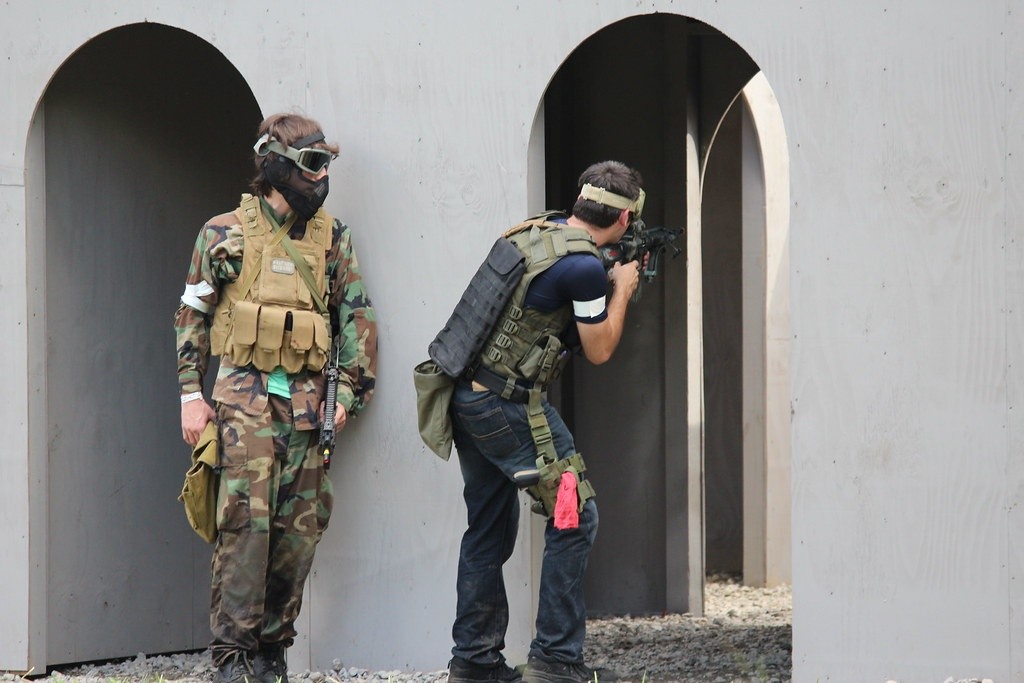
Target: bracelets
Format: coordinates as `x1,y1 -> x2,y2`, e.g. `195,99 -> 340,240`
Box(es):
180,391 -> 204,404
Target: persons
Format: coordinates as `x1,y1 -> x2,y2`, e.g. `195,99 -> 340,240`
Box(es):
174,111 -> 380,683
428,160 -> 650,683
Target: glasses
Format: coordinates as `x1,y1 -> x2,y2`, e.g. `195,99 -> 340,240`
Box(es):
263,139 -> 332,174
638,189 -> 644,214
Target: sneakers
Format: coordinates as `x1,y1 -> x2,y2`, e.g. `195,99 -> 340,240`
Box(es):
522,656 -> 618,683
213,649 -> 264,683
447,656 -> 522,683
252,642 -> 288,683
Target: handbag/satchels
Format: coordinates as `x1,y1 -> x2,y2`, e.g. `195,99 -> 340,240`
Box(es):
413,360 -> 455,462
178,416 -> 220,544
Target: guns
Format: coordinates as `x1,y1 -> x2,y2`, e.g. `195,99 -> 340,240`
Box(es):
511,459 -> 557,491
599,217 -> 685,286
318,305 -> 339,474
